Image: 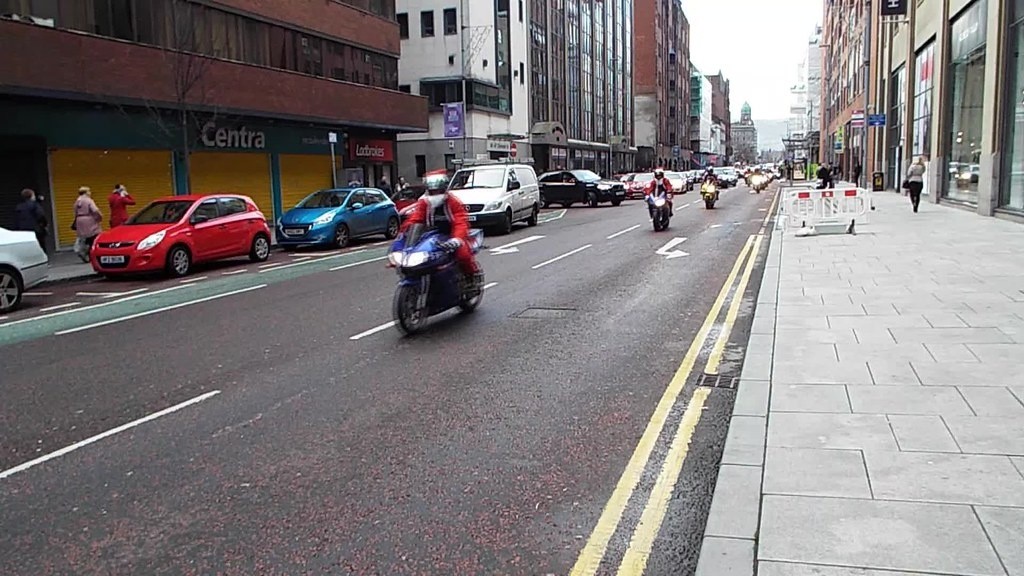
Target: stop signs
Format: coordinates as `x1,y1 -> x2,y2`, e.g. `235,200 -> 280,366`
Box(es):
511,144 -> 518,156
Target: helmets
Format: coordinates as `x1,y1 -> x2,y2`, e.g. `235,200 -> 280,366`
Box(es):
425,174 -> 449,190
706,166 -> 713,173
654,169 -> 664,179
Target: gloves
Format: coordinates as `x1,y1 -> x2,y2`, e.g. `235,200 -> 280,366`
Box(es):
121,190 -> 128,197
441,237 -> 461,248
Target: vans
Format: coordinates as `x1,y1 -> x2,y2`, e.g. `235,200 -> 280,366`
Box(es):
446,162 -> 542,234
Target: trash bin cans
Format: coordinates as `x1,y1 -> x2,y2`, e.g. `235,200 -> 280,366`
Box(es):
872,171 -> 883,191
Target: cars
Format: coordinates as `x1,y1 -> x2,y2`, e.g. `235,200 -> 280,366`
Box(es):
89,195 -> 271,277
536,169 -> 627,210
0,223 -> 50,318
613,163 -> 785,200
949,160 -> 979,186
274,185 -> 403,252
389,183 -> 427,216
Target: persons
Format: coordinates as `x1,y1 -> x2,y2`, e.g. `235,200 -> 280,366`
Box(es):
701,166 -> 720,200
15,189 -> 52,268
744,169 -> 761,189
107,184 -> 137,227
644,168 -> 673,222
907,157 -> 926,213
393,174 -> 479,292
854,161 -> 861,187
73,186 -> 103,263
816,162 -> 833,177
347,173 -> 410,199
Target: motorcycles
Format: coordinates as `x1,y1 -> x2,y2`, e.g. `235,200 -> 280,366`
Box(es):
700,180 -> 719,209
645,189 -> 672,231
382,223 -> 490,338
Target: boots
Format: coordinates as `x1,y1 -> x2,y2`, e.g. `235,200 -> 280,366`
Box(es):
79,245 -> 90,263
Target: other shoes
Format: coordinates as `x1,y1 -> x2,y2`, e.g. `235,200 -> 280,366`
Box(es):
914,204 -> 917,212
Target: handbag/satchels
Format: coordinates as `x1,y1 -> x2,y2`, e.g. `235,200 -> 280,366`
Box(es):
74,237 -> 86,253
903,179 -> 909,189
71,220 -> 78,230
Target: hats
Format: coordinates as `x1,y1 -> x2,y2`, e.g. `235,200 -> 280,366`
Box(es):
114,183 -> 127,195
